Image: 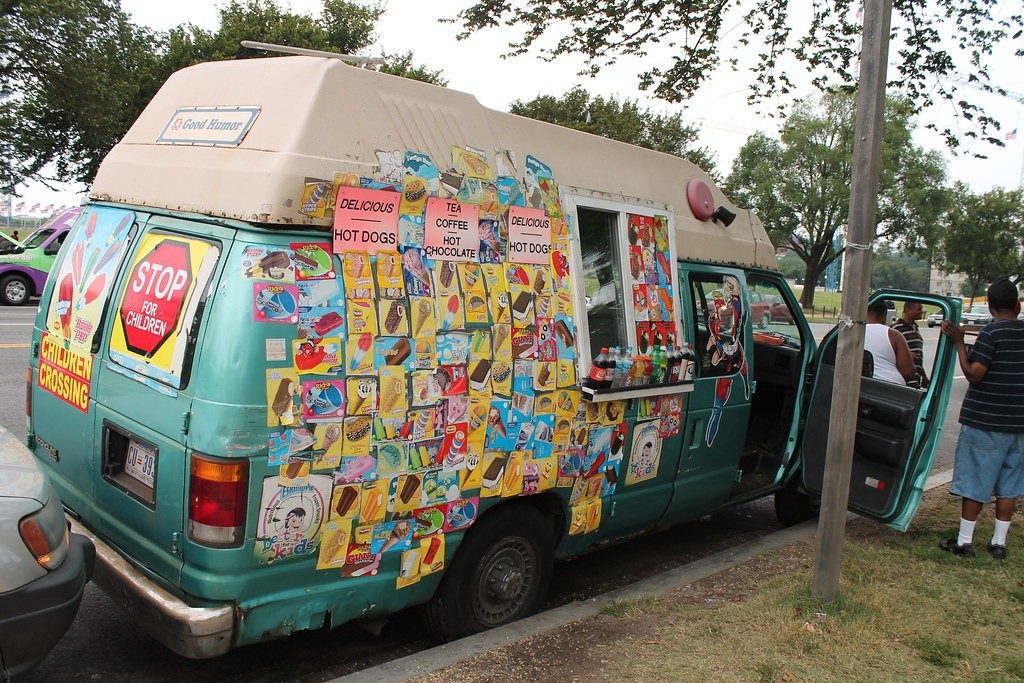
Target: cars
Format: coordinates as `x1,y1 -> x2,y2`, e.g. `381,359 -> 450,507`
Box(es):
692,285 -> 801,330
927,304 -> 1024,334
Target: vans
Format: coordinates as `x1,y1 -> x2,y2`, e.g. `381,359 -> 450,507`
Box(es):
1,422 -> 97,682
31,40 -> 966,661
1,207 -> 84,308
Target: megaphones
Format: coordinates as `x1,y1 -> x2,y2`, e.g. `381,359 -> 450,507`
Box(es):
714,206 -> 737,228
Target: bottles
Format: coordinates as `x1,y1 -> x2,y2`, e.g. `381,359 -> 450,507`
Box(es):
583,341 -> 695,401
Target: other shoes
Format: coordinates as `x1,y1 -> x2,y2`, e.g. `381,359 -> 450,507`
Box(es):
938,534 -> 976,557
987,538 -> 1006,559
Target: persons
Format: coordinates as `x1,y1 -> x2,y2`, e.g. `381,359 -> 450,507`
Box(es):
888,299 -> 930,388
11,230 -> 18,241
939,277 -> 1024,558
862,299 -> 915,385
